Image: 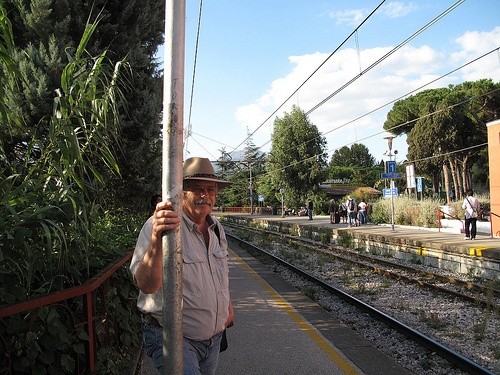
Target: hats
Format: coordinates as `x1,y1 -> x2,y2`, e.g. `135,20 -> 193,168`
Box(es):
183,157 -> 235,189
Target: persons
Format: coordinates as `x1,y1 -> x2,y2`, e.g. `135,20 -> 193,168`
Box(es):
329,195 -> 368,227
462,191 -> 480,240
298,207 -> 307,216
150,195 -> 162,211
130,157 -> 233,375
308,199 -> 313,220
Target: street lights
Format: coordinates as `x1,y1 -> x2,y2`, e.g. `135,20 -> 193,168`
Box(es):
382,136 -> 398,230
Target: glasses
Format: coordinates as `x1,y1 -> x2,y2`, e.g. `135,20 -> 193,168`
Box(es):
185,186 -> 218,197
